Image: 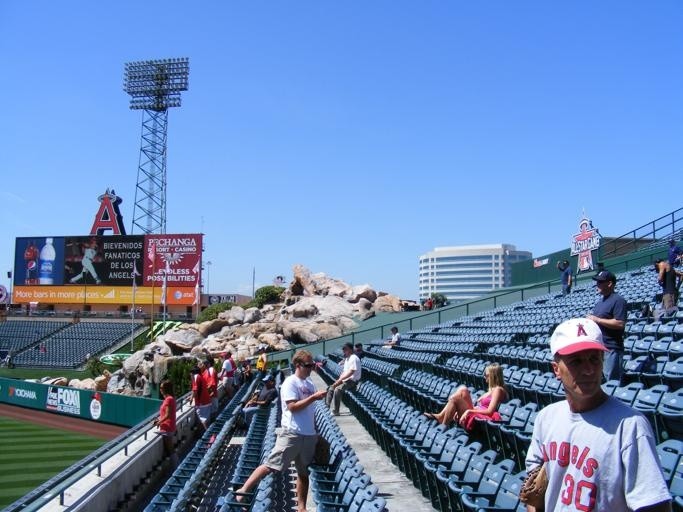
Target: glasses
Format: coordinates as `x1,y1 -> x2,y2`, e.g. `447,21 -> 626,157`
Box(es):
299,362 -> 314,368
484,373 -> 490,376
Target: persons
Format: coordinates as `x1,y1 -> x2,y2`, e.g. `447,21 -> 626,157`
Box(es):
654,257 -> 683,310
288,277 -> 314,296
557,260 -> 573,298
667,239 -> 683,268
382,327 -> 402,349
519,317 -> 673,512
153,343 -> 366,512
423,362 -> 510,439
33,341 -> 47,353
398,297 -> 437,312
69,239 -> 102,285
585,270 -> 627,382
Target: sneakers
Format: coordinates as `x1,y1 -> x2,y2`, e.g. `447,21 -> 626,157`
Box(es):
330,410 -> 339,416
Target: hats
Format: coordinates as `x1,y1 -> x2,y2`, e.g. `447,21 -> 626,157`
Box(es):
258,347 -> 265,351
549,321 -> 610,358
189,367 -> 202,373
355,343 -> 363,346
593,272 -> 615,283
563,261 -> 568,264
262,375 -> 275,381
668,239 -> 676,243
653,258 -> 662,274
343,343 -> 353,350
201,358 -> 212,363
219,352 -> 228,357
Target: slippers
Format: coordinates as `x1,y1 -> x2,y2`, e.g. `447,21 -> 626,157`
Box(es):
424,412 -> 440,424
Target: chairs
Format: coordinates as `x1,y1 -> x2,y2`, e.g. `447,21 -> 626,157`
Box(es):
311,257 -> 682,511
142,361 -> 388,512
0,318 -> 142,371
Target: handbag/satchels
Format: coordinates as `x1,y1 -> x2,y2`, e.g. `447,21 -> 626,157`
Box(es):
315,436 -> 330,466
